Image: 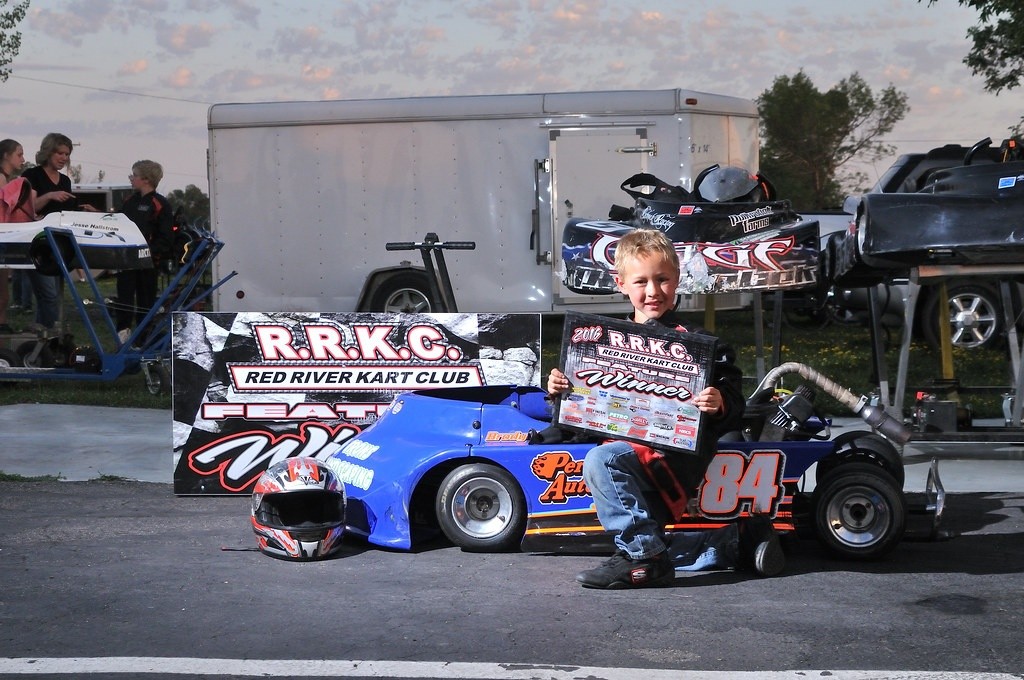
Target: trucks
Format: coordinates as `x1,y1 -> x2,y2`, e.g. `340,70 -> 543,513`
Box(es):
70,182 -> 139,214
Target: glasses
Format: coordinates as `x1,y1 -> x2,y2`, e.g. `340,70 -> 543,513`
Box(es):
129,175 -> 142,182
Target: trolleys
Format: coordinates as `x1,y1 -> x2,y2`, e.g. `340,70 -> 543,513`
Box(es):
0,226 -> 239,398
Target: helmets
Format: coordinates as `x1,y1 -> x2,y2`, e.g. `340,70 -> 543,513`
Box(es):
249,457 -> 348,561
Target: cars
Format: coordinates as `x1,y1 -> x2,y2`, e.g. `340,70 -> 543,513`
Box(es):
770,214 -> 1023,355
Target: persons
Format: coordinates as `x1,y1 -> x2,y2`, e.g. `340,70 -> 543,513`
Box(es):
80,159 -> 178,342
546,224 -> 747,592
0,137 -> 33,309
22,131 -> 78,325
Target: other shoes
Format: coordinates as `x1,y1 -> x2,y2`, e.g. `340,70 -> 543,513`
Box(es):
118,327 -> 133,344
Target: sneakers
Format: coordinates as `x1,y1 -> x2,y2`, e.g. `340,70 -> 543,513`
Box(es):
739,510 -> 787,576
576,549 -> 675,589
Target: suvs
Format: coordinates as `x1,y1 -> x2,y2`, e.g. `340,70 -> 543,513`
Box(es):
843,136 -> 1024,351
